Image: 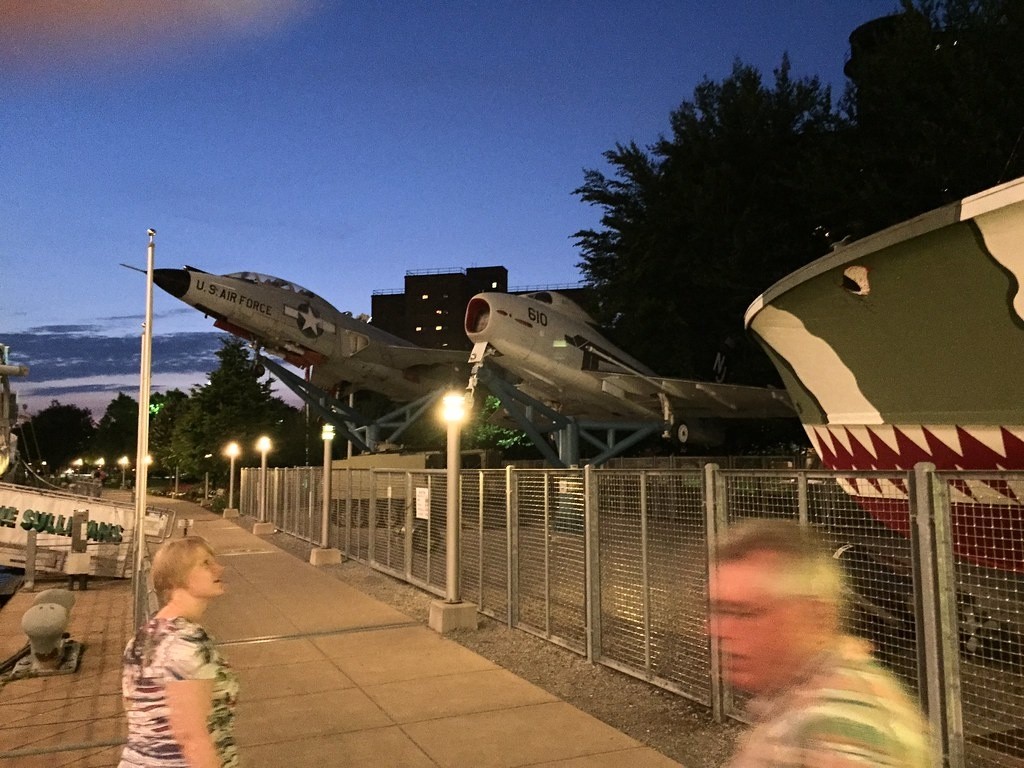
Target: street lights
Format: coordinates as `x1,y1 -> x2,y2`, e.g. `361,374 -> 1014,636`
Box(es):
437,392 -> 468,605
200,453 -> 213,506
320,420 -> 335,551
120,456 -> 127,491
223,442 -> 239,519
133,228 -> 157,631
253,436 -> 274,536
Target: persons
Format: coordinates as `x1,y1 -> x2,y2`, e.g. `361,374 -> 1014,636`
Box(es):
130,475 -> 136,501
710,516 -> 940,768
115,537 -> 240,768
74,469 -> 80,474
93,469 -> 107,502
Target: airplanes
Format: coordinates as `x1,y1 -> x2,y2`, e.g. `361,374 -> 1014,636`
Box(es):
116,259 -> 795,459
